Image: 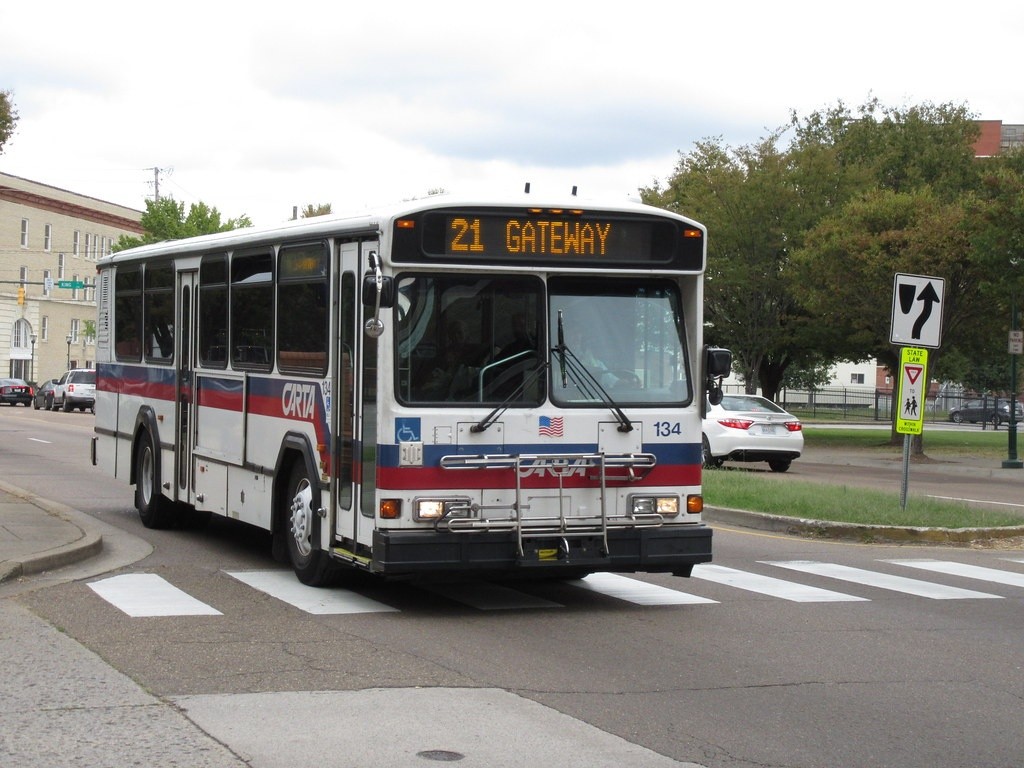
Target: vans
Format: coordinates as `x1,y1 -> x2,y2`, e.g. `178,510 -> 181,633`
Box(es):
950,398 -> 1022,426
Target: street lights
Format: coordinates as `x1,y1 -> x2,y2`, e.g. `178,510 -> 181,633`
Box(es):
30,335 -> 37,382
66,336 -> 73,371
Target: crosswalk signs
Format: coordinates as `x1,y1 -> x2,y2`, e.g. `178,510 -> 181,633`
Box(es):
899,363 -> 925,420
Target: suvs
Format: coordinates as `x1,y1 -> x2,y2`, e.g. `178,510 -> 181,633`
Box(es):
53,369 -> 96,413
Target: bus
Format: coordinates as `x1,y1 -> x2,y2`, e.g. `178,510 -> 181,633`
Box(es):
93,201 -> 731,603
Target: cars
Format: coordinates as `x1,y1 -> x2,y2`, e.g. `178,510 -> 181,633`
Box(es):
0,379 -> 33,408
34,380 -> 60,411
696,393 -> 805,472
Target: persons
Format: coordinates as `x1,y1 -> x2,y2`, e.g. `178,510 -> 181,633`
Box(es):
436,313 -> 641,391
